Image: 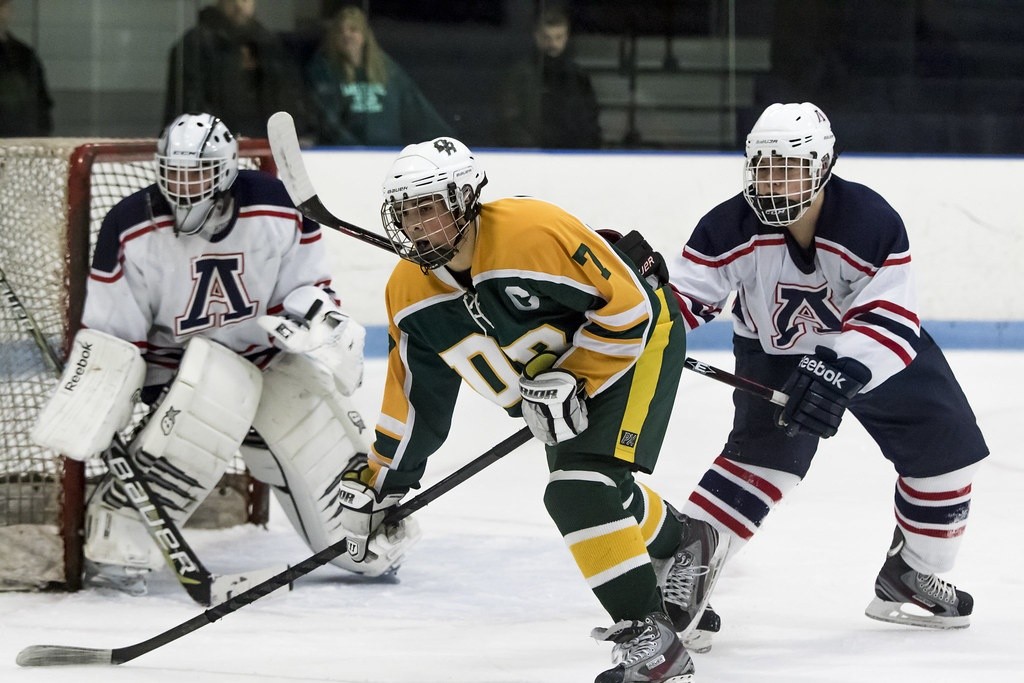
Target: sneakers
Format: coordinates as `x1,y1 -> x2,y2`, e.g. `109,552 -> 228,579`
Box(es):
681,602 -> 721,653
589,585 -> 697,683
82,559 -> 150,596
860,523 -> 975,631
661,499 -> 731,636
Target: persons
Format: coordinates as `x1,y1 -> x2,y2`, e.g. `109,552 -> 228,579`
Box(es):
666,101 -> 991,652
159,0 -> 300,142
499,11 -> 605,151
310,8 -> 455,146
0,0 -> 56,138
28,113 -> 420,580
341,137 -> 730,683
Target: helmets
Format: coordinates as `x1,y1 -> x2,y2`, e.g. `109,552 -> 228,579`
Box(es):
743,101 -> 837,228
380,138 -> 487,270
157,111 -> 241,231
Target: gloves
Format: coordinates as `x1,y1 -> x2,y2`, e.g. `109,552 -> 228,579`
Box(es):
339,470 -> 408,571
613,229 -> 668,291
520,352 -> 591,446
777,343 -> 873,440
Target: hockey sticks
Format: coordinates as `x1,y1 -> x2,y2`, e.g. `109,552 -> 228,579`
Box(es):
265,104 -> 793,408
15,424 -> 535,669
0,269 -> 259,612
